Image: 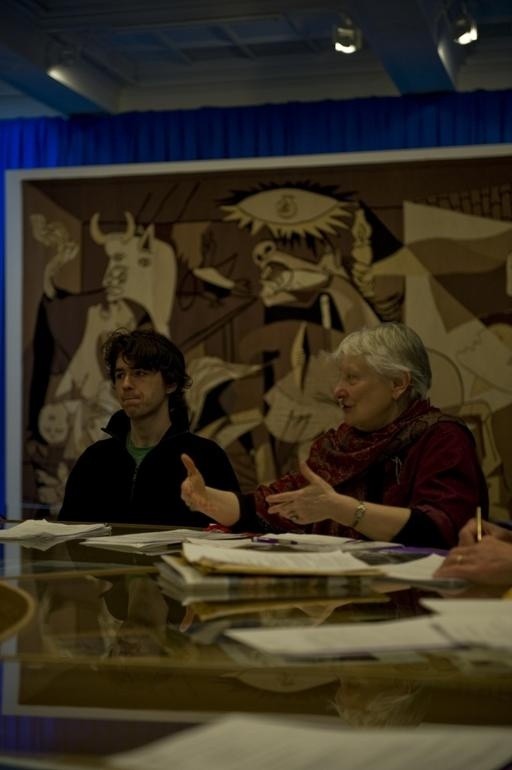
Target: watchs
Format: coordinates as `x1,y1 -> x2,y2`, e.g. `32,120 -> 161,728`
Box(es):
352,502 -> 366,528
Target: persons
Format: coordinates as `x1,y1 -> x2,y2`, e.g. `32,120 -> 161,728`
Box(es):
181,321 -> 489,550
67,539 -> 198,658
433,518 -> 512,587
57,327 -> 242,527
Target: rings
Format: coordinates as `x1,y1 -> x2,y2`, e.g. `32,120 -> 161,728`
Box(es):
292,516 -> 298,522
457,554 -> 463,563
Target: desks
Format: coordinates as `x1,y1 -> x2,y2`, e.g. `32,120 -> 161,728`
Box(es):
0,518 -> 512,769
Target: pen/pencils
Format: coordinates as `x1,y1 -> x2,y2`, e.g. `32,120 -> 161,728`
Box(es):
251,536 -> 298,544
476,507 -> 482,541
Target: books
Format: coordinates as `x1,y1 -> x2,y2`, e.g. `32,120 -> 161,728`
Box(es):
158,578 -> 372,607
158,554 -> 371,591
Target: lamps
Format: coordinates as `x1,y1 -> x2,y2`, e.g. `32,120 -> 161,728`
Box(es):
330,11 -> 366,54
48,44 -> 81,74
443,8 -> 479,49
